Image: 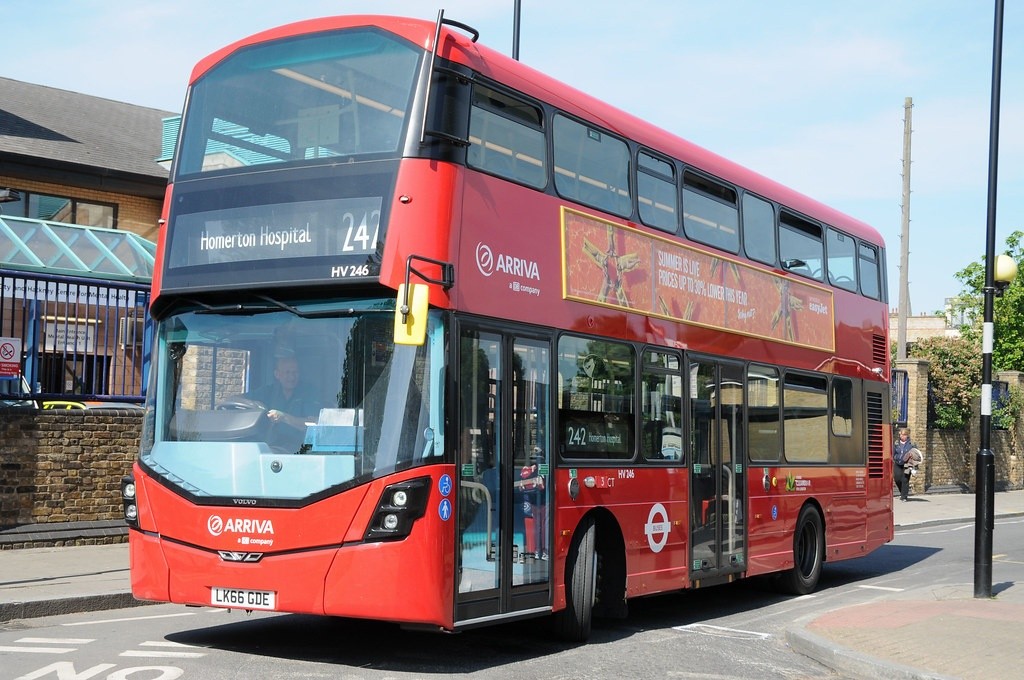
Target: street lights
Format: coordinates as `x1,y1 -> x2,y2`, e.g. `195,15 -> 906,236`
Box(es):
973,1 -> 1018,599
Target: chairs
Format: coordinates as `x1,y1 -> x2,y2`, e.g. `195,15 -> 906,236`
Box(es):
784,260 -> 856,292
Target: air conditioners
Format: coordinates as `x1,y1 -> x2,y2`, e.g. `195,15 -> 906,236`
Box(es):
118,316 -> 144,347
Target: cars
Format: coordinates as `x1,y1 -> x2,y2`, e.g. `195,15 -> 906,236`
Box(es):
0,375 -> 142,410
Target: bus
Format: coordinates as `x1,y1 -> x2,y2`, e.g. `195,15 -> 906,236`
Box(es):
120,12 -> 895,643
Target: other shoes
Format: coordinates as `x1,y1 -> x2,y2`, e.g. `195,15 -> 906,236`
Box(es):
903,498 -> 907,502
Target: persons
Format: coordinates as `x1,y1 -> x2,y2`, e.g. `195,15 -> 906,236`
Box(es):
215,357 -> 319,454
893,428 -> 924,502
